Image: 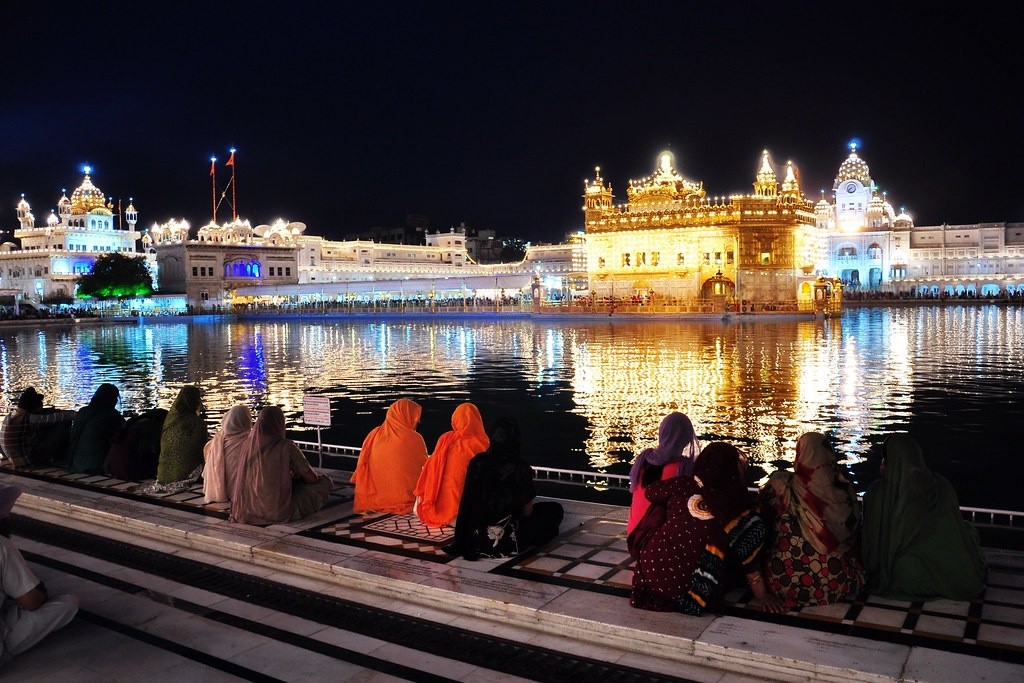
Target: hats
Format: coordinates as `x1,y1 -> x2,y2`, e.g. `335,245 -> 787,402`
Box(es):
18,387 -> 43,408
0,482 -> 23,518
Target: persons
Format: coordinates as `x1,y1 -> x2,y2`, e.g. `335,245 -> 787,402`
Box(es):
455,415 -> 564,558
579,294 -> 650,306
190,305 -> 193,315
844,288 -> 1024,300
756,433 -> 866,606
200,404 -> 251,504
212,304 -> 222,314
626,412 -> 695,535
67,383 -> 125,474
349,399 -> 429,516
629,442 -> 786,617
156,385 -> 209,485
413,402 -> 491,526
860,433 -> 988,600
0,387 -> 78,468
102,409 -> 169,481
233,297 -> 516,310
0,535 -> 79,666
227,406 -> 334,525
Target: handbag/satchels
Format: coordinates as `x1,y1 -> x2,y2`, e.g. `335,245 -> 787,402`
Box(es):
767,473 -> 866,609
472,515 -> 518,559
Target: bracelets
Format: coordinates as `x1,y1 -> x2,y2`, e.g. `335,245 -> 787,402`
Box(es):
747,573 -> 762,585
